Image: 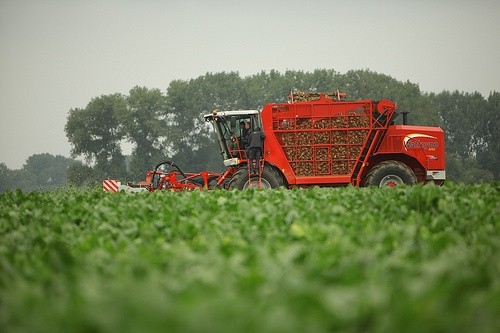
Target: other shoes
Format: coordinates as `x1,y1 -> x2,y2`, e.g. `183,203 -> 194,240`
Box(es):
256,172 -> 259,174
251,172 -> 254,174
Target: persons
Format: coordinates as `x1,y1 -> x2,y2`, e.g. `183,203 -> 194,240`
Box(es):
237,120 -> 248,155
248,126 -> 266,178
242,122 -> 254,157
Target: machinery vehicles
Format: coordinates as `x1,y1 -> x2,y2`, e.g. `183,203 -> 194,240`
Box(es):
135,89 -> 448,193
